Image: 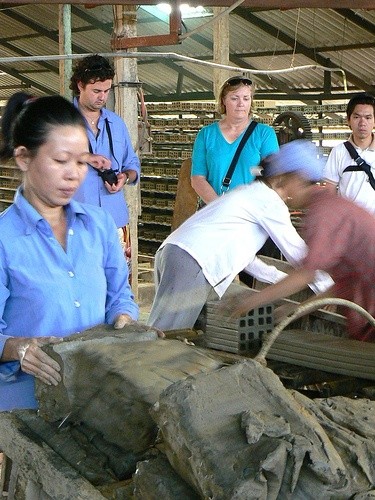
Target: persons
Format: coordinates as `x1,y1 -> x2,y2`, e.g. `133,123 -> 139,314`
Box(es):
0,91 -> 167,413
147,93 -> 375,345
67,54 -> 140,258
190,75 -> 280,330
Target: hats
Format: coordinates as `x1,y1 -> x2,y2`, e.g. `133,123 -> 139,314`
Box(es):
267,141 -> 325,180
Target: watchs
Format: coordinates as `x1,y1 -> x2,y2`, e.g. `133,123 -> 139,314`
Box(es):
122,171 -> 129,186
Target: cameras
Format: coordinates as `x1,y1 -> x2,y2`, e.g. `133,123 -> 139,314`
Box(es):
101,169 -> 117,186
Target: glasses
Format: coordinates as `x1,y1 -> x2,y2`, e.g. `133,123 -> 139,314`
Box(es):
222,78 -> 253,92
83,63 -> 112,72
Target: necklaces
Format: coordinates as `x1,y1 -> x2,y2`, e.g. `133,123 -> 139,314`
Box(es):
90,116 -> 98,124
